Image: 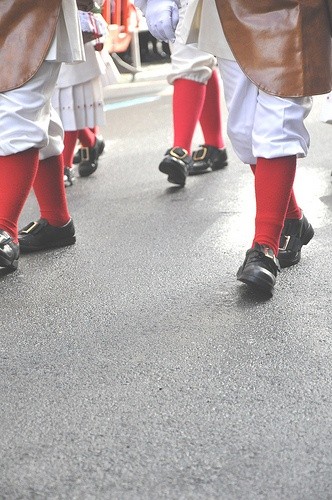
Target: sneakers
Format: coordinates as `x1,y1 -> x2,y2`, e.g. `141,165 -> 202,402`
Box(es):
72,149 -> 81,164
18,218 -> 76,251
236,242 -> 281,297
0,229 -> 20,269
159,146 -> 190,185
63,166 -> 73,187
78,137 -> 105,177
277,212 -> 314,268
187,144 -> 228,175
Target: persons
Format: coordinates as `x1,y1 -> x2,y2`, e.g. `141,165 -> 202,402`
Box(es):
134,0 -> 228,185
51,0 -> 122,188
0,0 -> 86,269
177,0 -> 314,291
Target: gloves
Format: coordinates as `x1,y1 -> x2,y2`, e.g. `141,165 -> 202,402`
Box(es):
146,0 -> 180,44
